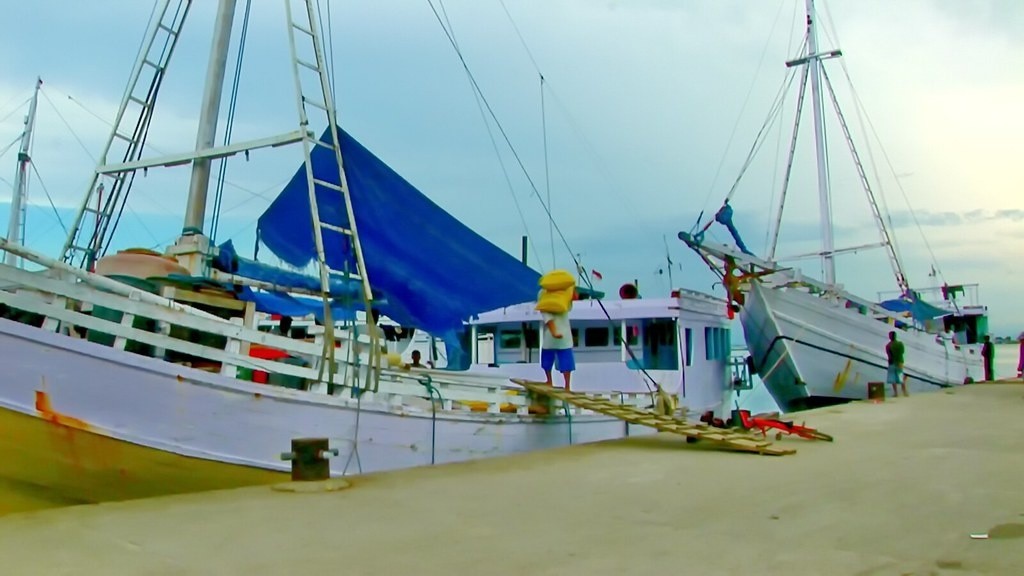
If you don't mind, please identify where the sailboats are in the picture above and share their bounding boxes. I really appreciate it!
[677,0,991,413]
[0,0,747,504]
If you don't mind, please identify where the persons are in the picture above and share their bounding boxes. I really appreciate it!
[981,336,995,382]
[405,350,427,369]
[269,316,293,338]
[535,271,578,392]
[885,331,908,397]
